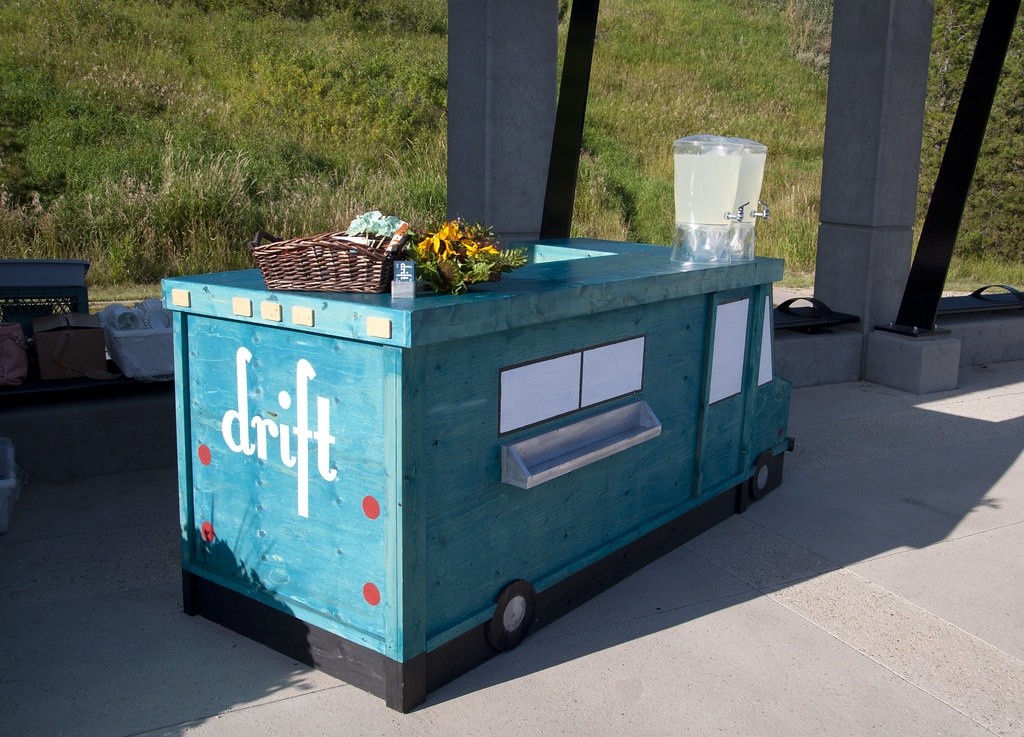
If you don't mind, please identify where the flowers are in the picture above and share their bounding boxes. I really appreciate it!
[386,210,529,298]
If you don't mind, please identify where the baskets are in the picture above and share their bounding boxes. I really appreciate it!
[0,438,15,533]
[247,230,415,293]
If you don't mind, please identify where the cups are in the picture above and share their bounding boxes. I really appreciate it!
[108,304,140,330]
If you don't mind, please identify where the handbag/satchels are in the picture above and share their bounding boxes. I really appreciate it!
[0,317,31,387]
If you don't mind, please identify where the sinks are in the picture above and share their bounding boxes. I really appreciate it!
[512,240,618,271]
[737,202,750,222]
[758,200,768,219]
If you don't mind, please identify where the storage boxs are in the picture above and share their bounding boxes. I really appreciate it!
[96,312,173,379]
[32,317,107,380]
